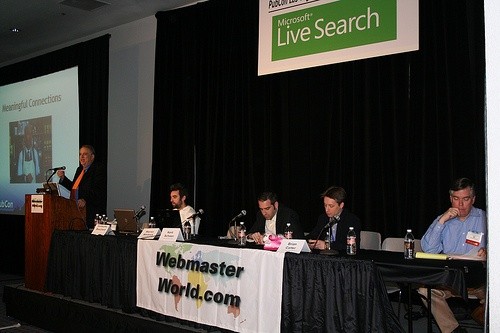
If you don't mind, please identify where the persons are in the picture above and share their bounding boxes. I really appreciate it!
[17,126,40,183]
[309,186,351,251]
[58,145,99,228]
[418,177,489,333]
[246,193,300,243]
[169,184,200,235]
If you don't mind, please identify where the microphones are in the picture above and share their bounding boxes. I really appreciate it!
[186,209,204,219]
[324,216,340,229]
[232,210,247,221]
[133,205,146,218]
[49,166,66,171]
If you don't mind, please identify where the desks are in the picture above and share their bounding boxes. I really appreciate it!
[44,228,487,333]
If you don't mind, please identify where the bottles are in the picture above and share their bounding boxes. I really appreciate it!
[346,227,356,255]
[404,230,416,259]
[94,214,108,227]
[183,220,191,241]
[148,217,155,228]
[238,222,246,245]
[284,224,293,239]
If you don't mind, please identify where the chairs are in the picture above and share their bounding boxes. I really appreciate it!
[360,231,489,333]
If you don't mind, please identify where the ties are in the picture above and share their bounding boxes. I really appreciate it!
[72,167,85,190]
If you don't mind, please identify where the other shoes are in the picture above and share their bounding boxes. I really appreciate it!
[454,328,467,333]
